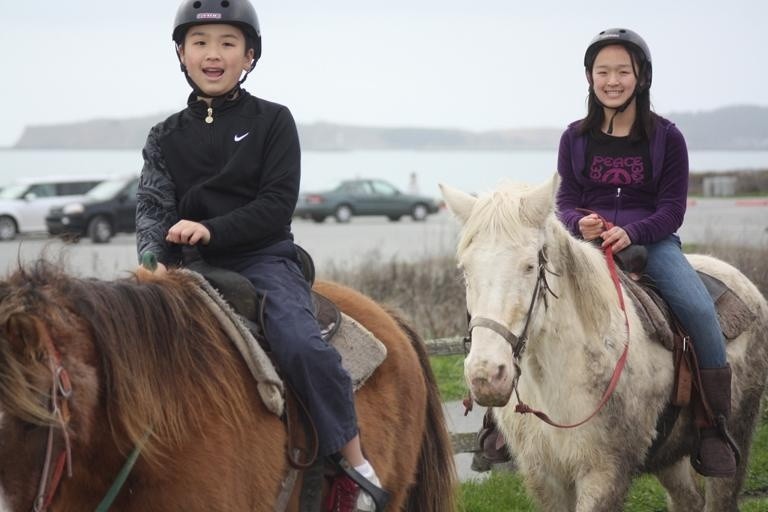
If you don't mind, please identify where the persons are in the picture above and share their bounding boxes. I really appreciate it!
[133,0,387,511]
[478,27,738,478]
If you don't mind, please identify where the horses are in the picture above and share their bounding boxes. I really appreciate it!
[0,227,463,512]
[436,171,768,512]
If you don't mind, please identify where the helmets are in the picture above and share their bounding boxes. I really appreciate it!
[584,28,653,94]
[172,0,262,63]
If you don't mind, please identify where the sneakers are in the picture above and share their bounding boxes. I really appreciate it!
[328,460,380,512]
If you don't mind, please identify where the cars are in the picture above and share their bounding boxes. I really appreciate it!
[44,176,140,244]
[0,174,111,241]
[291,179,446,223]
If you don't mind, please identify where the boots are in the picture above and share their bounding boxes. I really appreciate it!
[690,361,738,478]
[484,423,513,463]
